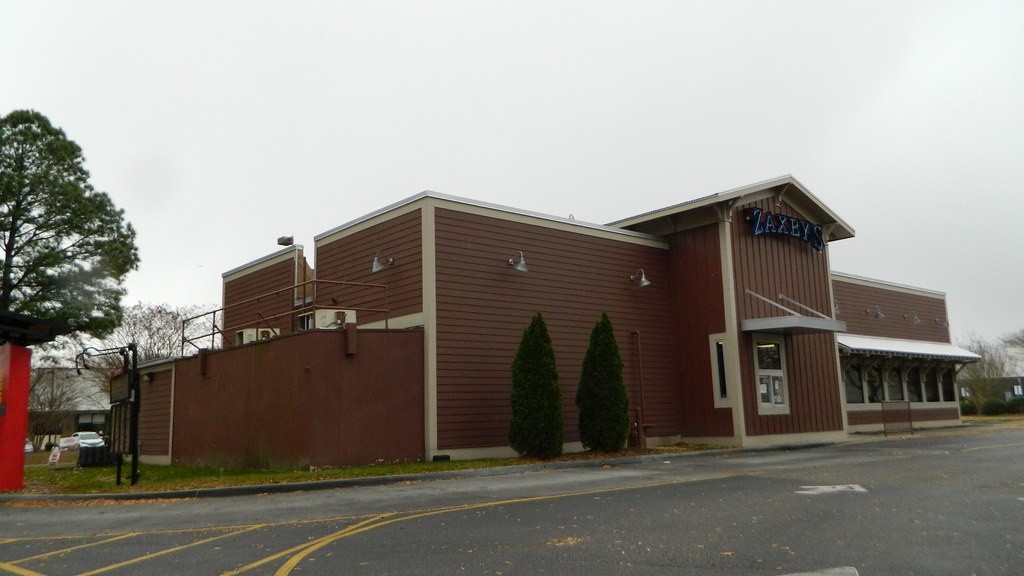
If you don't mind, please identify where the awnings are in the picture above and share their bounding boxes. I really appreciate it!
[836,336,981,383]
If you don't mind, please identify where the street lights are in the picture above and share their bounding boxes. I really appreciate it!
[76,343,140,485]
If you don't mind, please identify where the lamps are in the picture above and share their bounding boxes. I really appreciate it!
[867,305,885,319]
[509,249,529,272]
[372,250,394,273]
[903,311,921,324]
[934,316,949,327]
[629,268,652,287]
[833,298,840,314]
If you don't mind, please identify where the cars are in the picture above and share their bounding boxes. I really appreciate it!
[72,432,105,447]
[24,438,34,453]
